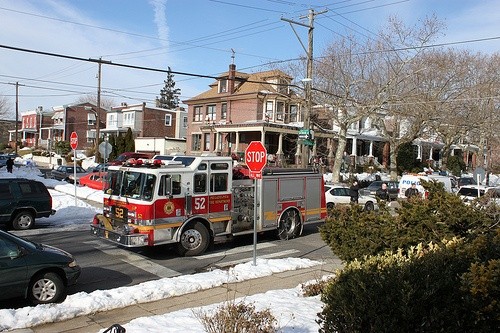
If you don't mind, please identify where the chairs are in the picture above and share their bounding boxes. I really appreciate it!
[173,182,180,194]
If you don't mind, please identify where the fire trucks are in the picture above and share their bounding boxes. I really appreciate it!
[89,154,328,255]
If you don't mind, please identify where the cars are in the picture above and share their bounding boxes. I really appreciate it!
[49,165,86,184]
[0,230,81,304]
[80,173,109,192]
[369,180,399,200]
[457,177,476,188]
[324,184,377,214]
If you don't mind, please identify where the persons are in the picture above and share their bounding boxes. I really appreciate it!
[405,183,419,199]
[230,152,246,162]
[123,181,155,197]
[6,156,14,173]
[308,155,335,166]
[349,179,359,205]
[376,183,391,210]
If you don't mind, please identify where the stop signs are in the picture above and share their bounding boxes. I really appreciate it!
[69,132,78,150]
[244,141,266,179]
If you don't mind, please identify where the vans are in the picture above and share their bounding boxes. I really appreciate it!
[398,175,452,200]
[455,184,500,210]
[0,178,56,229]
